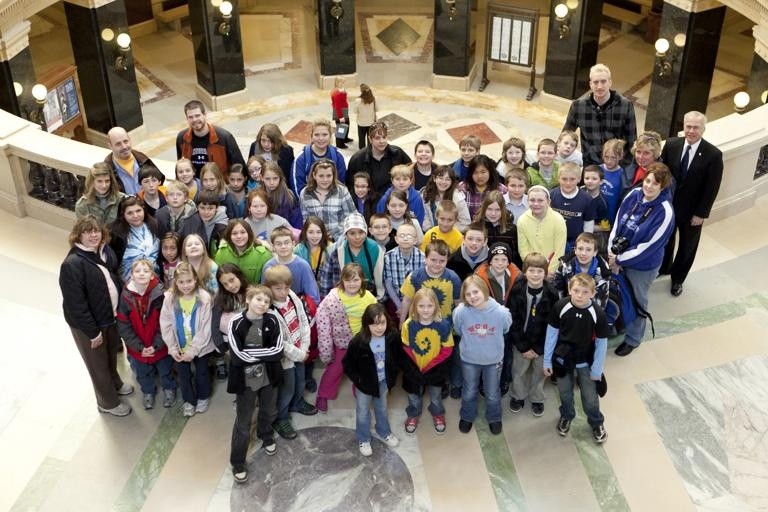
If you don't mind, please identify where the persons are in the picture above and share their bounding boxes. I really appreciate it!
[211,263,250,379]
[556,130,583,169]
[400,288,457,437]
[180,189,231,259]
[330,75,355,150]
[382,222,430,333]
[353,83,379,150]
[298,157,358,244]
[262,264,319,441]
[115,259,179,410]
[175,157,202,203]
[366,213,397,252]
[447,222,492,399]
[225,163,251,218]
[319,211,396,393]
[342,302,425,458]
[134,166,169,218]
[103,126,165,196]
[260,226,321,393]
[623,131,661,188]
[348,170,381,239]
[226,284,285,484]
[517,185,569,287]
[472,190,521,270]
[290,120,347,229]
[159,232,183,291]
[452,274,514,436]
[554,232,611,314]
[159,262,214,418]
[213,218,273,285]
[259,161,297,221]
[474,241,524,400]
[582,166,611,263]
[606,162,676,357]
[505,252,559,416]
[448,134,481,183]
[596,137,627,228]
[59,214,135,417]
[181,233,228,380]
[70,124,92,145]
[525,138,561,194]
[397,238,463,398]
[73,162,129,227]
[543,273,610,444]
[292,215,334,292]
[176,100,248,186]
[655,111,724,297]
[554,64,637,189]
[500,168,531,226]
[246,154,267,191]
[409,139,442,192]
[154,182,209,257]
[418,164,471,237]
[314,264,380,414]
[495,138,531,186]
[460,154,508,223]
[344,121,413,198]
[549,161,596,257]
[192,162,234,220]
[376,164,425,229]
[371,190,424,254]
[420,199,465,257]
[102,194,166,294]
[243,189,292,246]
[249,123,294,191]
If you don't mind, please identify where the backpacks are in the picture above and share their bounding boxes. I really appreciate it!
[605,271,655,340]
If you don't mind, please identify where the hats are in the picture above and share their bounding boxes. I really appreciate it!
[343,210,367,235]
[487,242,512,265]
[528,184,551,200]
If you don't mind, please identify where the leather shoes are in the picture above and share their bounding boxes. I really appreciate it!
[670,278,684,297]
[615,341,639,357]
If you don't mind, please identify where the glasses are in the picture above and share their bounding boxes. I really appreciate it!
[82,229,102,235]
[250,166,262,175]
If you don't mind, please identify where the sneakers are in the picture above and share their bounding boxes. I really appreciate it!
[97,402,132,417]
[143,392,155,410]
[376,430,400,447]
[305,379,317,392]
[591,422,608,443]
[557,415,571,437]
[231,464,248,483]
[316,392,328,414]
[405,417,419,433]
[216,361,227,382]
[162,387,177,407]
[439,385,447,399]
[500,383,509,395]
[433,416,446,435]
[262,439,277,456]
[297,400,317,416]
[489,419,502,435]
[116,382,135,395]
[451,386,462,398]
[196,397,211,413]
[359,439,372,457]
[478,386,486,399]
[532,402,545,417]
[272,418,297,439]
[458,419,471,433]
[510,397,524,413]
[182,402,196,416]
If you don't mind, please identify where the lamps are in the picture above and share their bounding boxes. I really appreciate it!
[30,84,49,126]
[734,90,752,115]
[554,3,571,42]
[653,37,674,82]
[113,33,137,72]
[216,1,233,37]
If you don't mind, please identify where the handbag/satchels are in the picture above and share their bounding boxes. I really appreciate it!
[334,120,349,140]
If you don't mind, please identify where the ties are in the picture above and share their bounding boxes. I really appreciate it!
[680,144,692,176]
